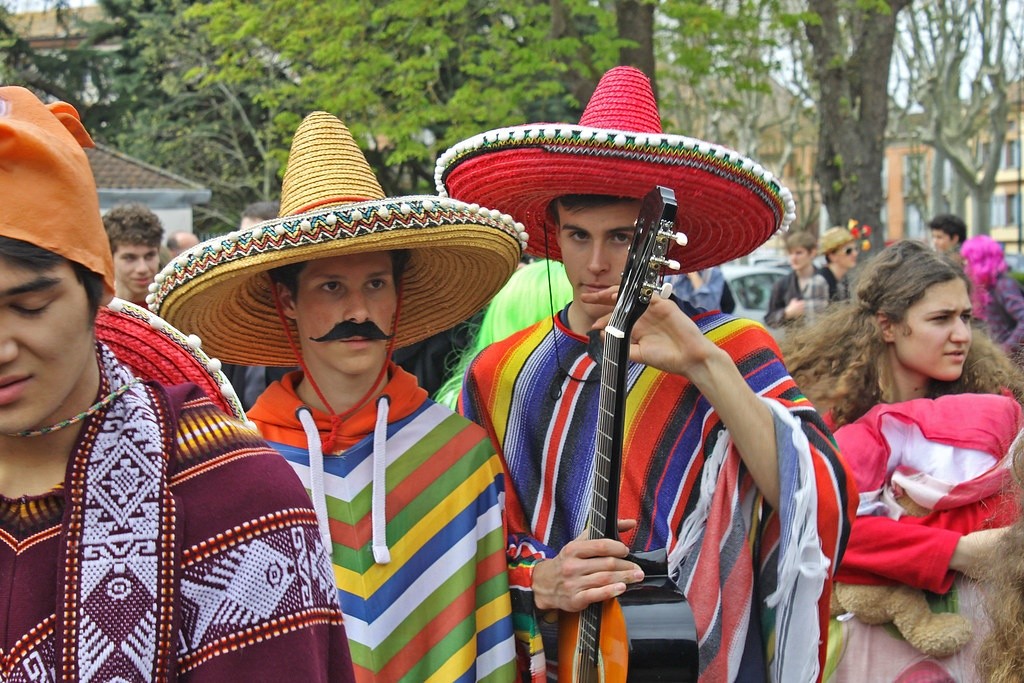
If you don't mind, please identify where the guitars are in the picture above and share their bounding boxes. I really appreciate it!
[555,186,700,683]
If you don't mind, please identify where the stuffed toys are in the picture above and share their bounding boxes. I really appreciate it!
[828,494,976,656]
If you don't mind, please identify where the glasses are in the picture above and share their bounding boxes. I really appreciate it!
[832,246,861,255]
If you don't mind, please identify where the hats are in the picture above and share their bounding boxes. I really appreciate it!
[435,66,796,281]
[0,87,116,304]
[816,227,853,255]
[146,111,528,368]
[96,296,254,432]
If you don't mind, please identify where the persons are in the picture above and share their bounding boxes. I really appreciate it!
[769,241,1024,683]
[665,269,726,311]
[100,196,163,309]
[167,231,201,259]
[434,65,859,683]
[961,233,1024,369]
[927,213,967,270]
[765,232,818,337]
[221,202,301,412]
[803,226,859,319]
[145,112,530,683]
[0,85,355,682]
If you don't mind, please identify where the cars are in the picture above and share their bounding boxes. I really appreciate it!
[719,253,796,342]
